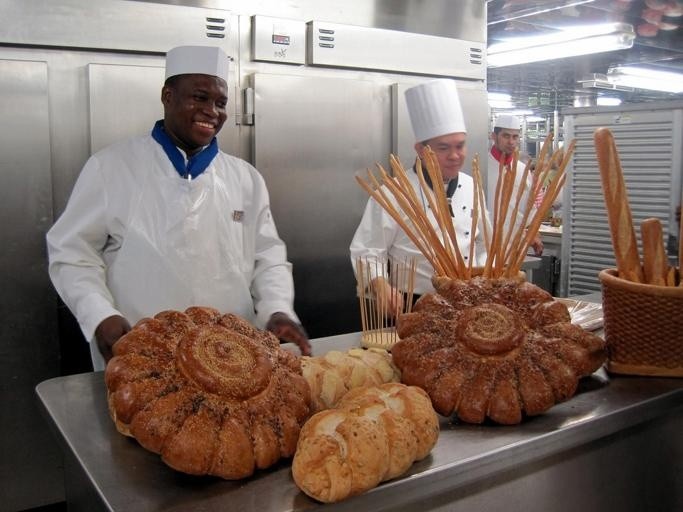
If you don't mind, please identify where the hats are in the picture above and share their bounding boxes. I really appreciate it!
[164,46,229,86]
[495,115,521,130]
[405,79,466,142]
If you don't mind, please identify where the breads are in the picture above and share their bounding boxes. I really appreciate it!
[291,383,441,503]
[665,267,679,285]
[392,274,611,427]
[300,347,402,413]
[105,307,315,481]
[640,218,668,286]
[594,128,645,285]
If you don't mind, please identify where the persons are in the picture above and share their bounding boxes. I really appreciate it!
[45,44,312,372]
[487,113,545,258]
[348,77,496,327]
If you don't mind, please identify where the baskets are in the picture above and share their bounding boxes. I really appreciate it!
[599,267,683,376]
